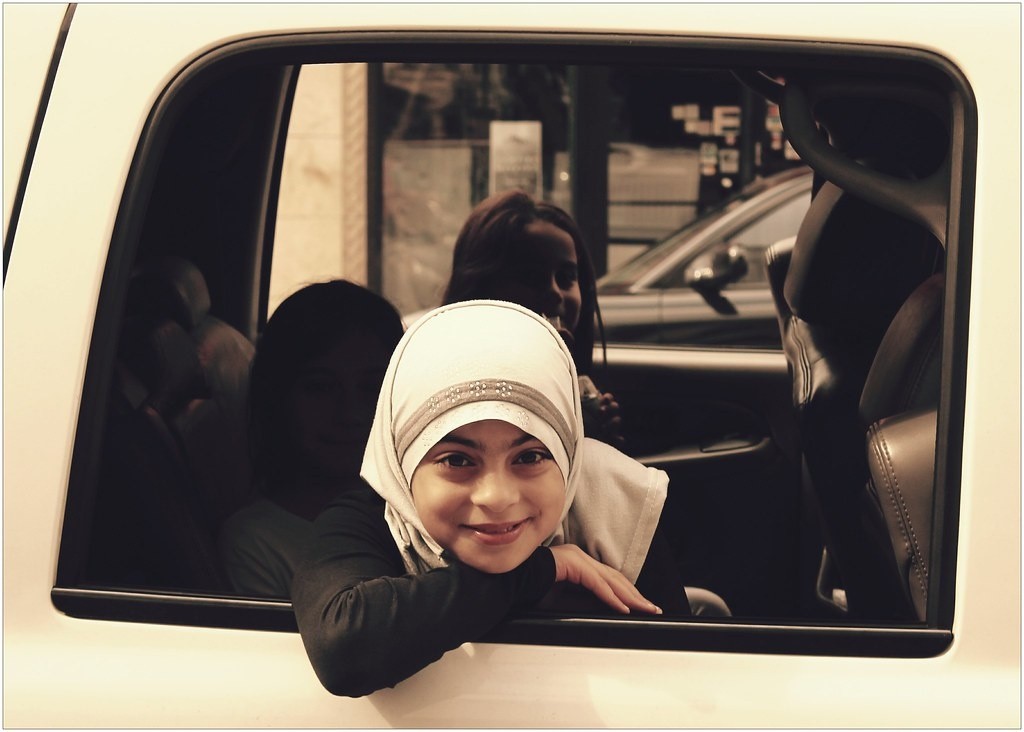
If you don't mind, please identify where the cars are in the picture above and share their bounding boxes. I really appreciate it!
[1,4,1022,728]
[594,159,817,350]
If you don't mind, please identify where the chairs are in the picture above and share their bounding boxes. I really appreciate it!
[782,180,947,634]
[101,240,260,592]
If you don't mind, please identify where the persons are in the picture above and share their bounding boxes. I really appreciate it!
[216,282,403,600]
[442,193,733,617]
[290,294,690,697]
